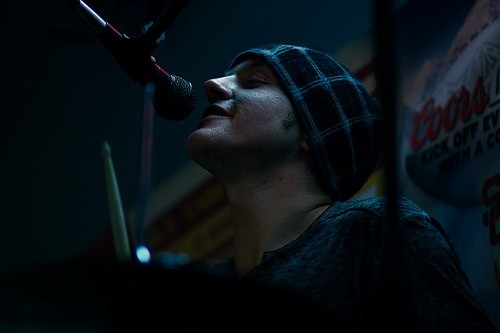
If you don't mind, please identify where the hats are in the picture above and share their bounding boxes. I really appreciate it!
[230,45,383,201]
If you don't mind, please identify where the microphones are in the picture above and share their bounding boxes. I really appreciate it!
[66,0,197,122]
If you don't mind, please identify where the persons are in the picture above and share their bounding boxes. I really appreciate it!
[129,43,494,333]
